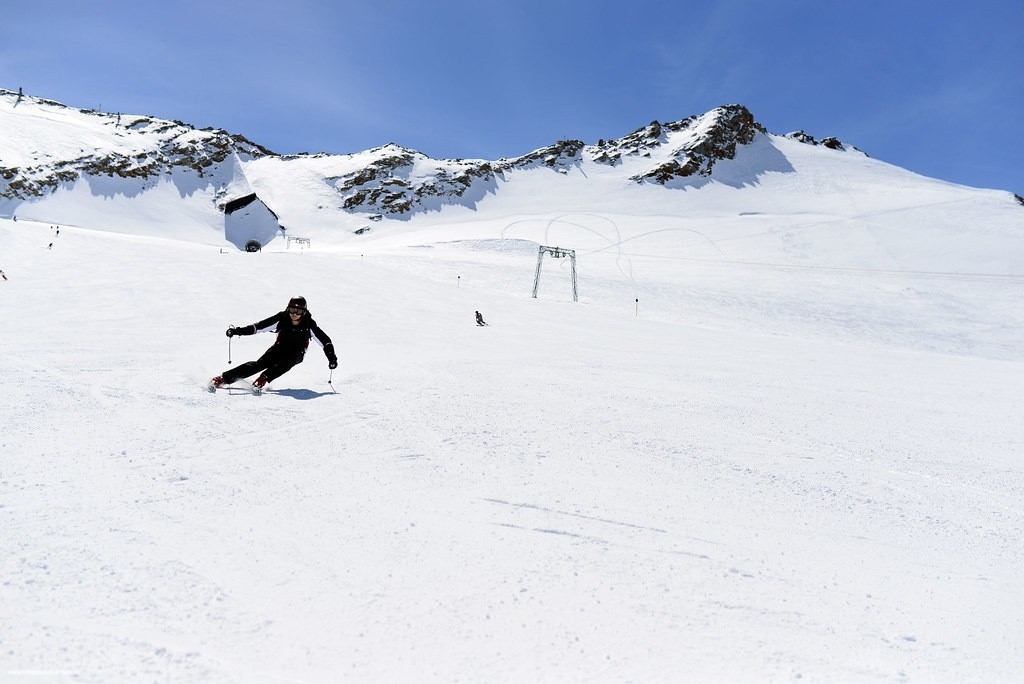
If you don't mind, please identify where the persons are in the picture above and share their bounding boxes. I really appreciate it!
[475,311,486,326]
[0,270,8,280]
[207,297,337,395]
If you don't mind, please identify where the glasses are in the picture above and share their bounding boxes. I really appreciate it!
[287,306,306,315]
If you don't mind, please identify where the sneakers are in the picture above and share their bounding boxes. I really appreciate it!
[252,374,267,389]
[212,374,224,388]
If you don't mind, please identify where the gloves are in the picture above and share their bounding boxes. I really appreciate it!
[323,344,338,369]
[226,324,257,337]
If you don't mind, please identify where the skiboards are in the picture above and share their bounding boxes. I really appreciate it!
[201,381,263,397]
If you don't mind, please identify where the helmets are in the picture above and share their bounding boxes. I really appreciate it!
[288,296,307,310]
[475,311,478,313]
